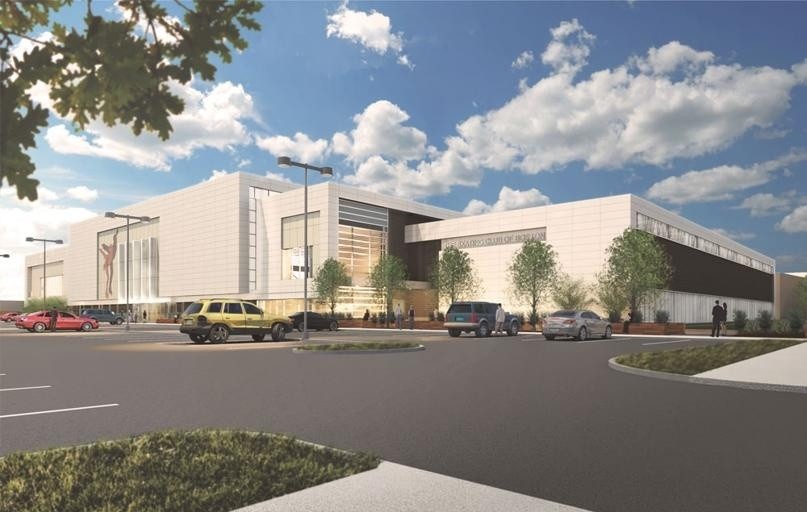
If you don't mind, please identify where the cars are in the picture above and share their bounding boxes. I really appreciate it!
[542,310,613,342]
[0,312,29,321]
[16,310,99,332]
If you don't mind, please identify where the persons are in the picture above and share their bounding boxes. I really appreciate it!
[407,304,415,330]
[432,306,438,321]
[111,308,147,323]
[363,309,370,321]
[626,313,631,321]
[98,233,118,298]
[711,299,723,337]
[721,302,727,336]
[493,303,505,336]
[49,306,58,331]
[395,304,403,330]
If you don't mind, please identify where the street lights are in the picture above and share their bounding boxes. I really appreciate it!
[103,211,150,330]
[25,236,63,308]
[277,155,334,342]
[0,254,10,258]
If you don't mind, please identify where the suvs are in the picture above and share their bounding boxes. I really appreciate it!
[178,298,294,344]
[81,308,125,325]
[288,311,339,332]
[443,300,522,339]
